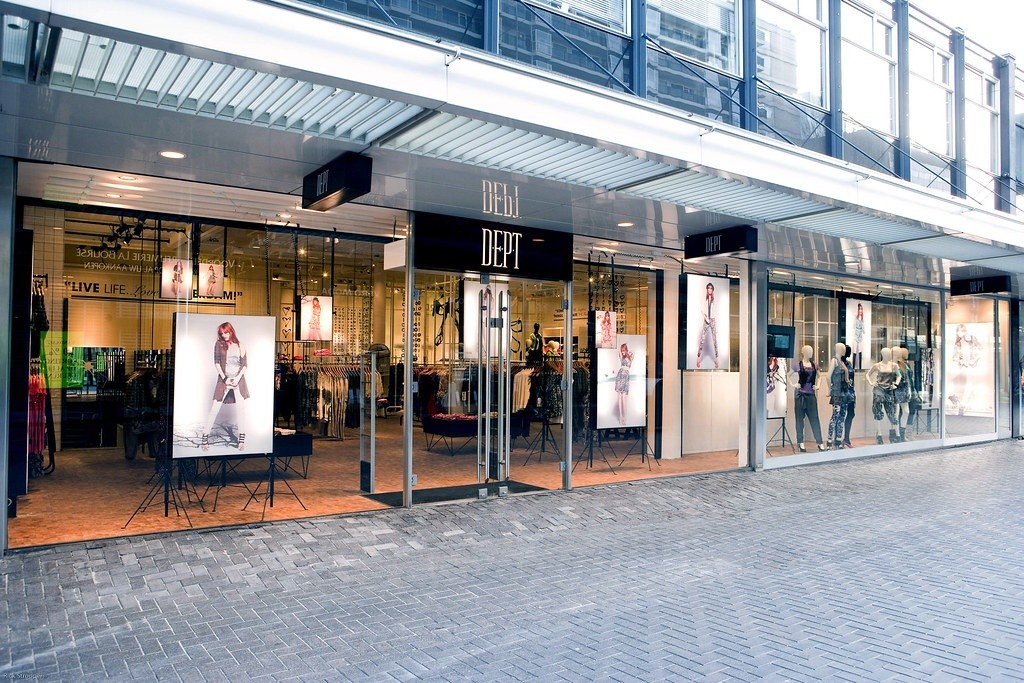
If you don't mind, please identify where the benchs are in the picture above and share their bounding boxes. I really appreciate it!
[194,430,313,487]
[423,411,499,458]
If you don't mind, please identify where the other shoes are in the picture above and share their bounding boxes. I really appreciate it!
[876,434,884,445]
[202,445,208,452]
[238,442,244,451]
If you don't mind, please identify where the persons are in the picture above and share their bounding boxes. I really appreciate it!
[614,343,634,425]
[948,324,982,410]
[890,346,919,441]
[853,303,865,369]
[786,345,825,452]
[207,265,217,296]
[482,285,494,357]
[172,260,183,297]
[865,348,902,445]
[202,322,251,452]
[697,283,719,368]
[826,343,856,451]
[307,297,322,340]
[601,311,612,348]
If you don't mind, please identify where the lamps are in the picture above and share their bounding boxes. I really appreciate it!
[77,216,146,253]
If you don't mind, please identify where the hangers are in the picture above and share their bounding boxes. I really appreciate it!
[31,274,45,297]
[29,359,45,384]
[297,358,588,377]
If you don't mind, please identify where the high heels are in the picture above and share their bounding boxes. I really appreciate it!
[798,443,806,452]
[818,445,825,451]
[827,438,855,449]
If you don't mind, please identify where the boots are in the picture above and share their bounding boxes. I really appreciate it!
[858,352,862,369]
[852,353,856,370]
[899,427,908,442]
[889,429,901,443]
